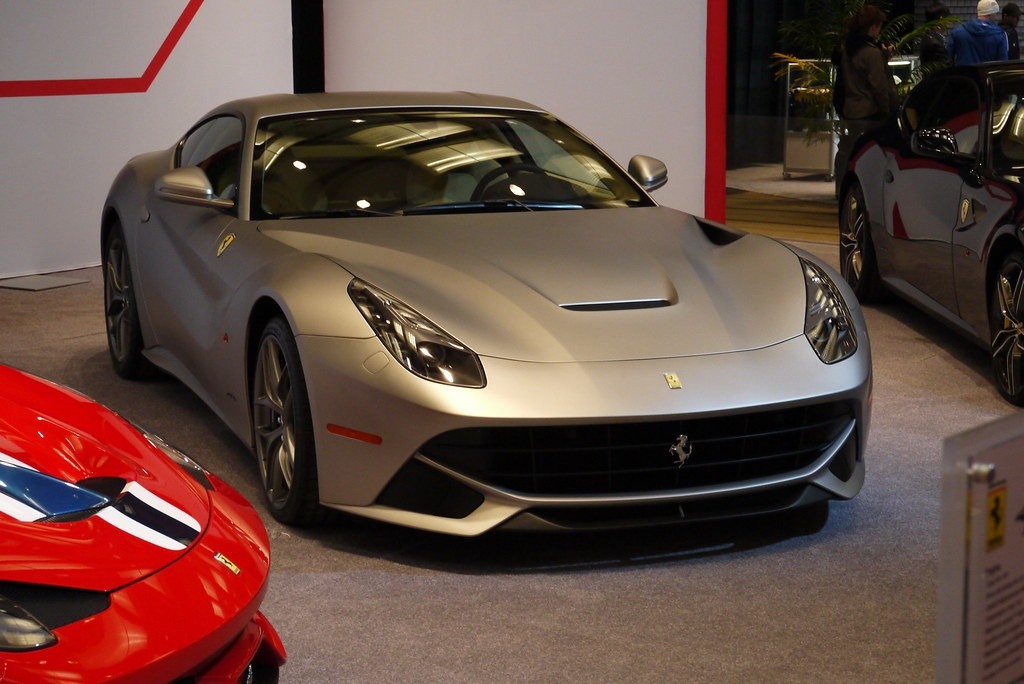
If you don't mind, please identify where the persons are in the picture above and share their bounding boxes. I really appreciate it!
[947,0,1009,65]
[998,2,1024,59]
[831,5,898,196]
[919,2,952,78]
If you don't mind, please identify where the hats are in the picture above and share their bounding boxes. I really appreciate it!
[977,0,999,16]
[1002,2,1024,17]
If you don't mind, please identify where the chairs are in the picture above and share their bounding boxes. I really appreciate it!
[263,157,321,214]
[406,155,477,205]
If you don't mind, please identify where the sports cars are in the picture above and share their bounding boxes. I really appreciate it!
[102,93,871,539]
[839,60,1024,408]
[0,364,287,684]
